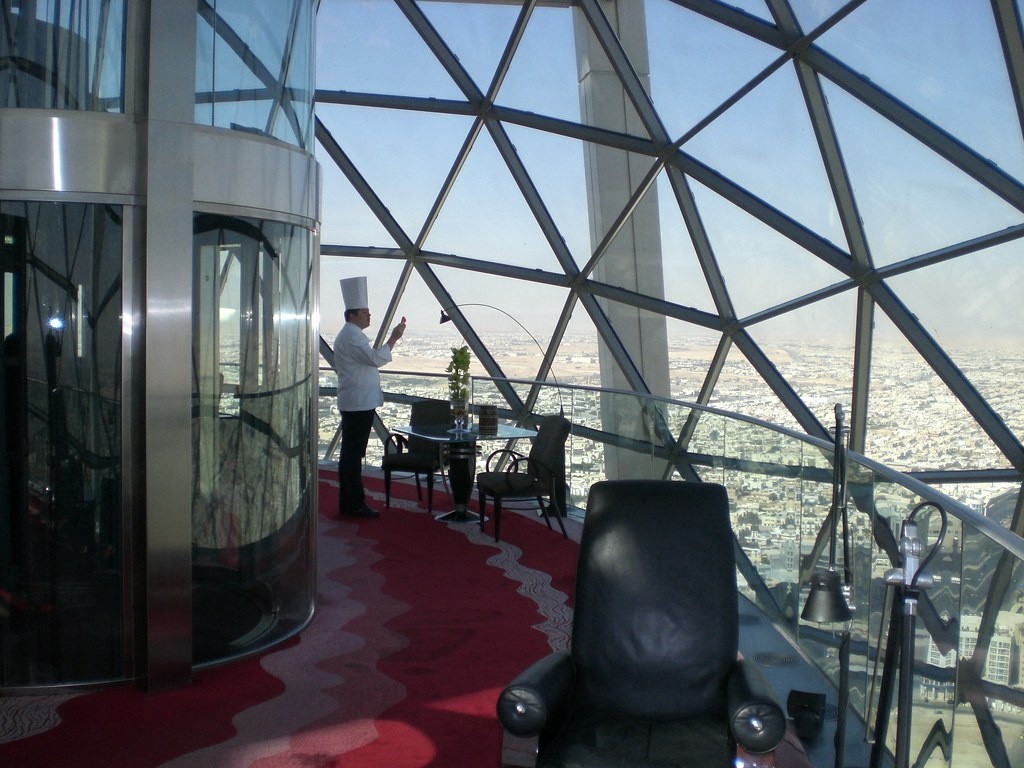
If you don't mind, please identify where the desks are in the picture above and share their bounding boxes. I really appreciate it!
[392,423,539,524]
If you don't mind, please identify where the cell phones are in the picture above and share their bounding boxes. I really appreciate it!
[401,317,406,326]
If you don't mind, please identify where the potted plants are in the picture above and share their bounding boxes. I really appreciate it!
[446,345,474,434]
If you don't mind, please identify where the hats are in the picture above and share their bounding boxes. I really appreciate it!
[340,276,368,310]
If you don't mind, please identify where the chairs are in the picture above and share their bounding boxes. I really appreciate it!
[477,416,572,543]
[496,478,786,768]
[381,401,451,513]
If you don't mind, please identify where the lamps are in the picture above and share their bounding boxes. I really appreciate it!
[799,403,857,768]
[441,303,566,418]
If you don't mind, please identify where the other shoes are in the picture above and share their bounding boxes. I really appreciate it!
[354,504,381,519]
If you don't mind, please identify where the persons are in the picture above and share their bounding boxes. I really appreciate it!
[334,277,406,519]
